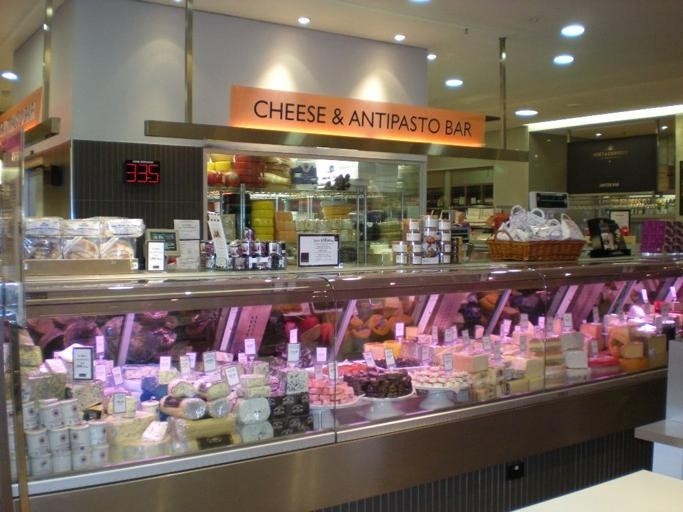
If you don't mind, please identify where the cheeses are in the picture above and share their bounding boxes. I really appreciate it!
[1,312,341,484]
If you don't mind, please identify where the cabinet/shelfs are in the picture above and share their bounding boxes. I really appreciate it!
[209,190,425,265]
[426,168,494,222]
[0,258,683,512]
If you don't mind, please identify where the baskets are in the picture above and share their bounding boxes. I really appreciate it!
[485,230,586,261]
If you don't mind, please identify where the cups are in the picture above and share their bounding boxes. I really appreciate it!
[8,432,16,454]
[24,427,49,457]
[10,461,17,483]
[41,402,65,430]
[39,398,58,425]
[53,449,72,474]
[22,400,38,430]
[69,424,90,445]
[141,400,160,420]
[60,399,82,425]
[49,428,70,450]
[26,456,30,476]
[72,447,92,471]
[6,401,14,433]
[30,453,53,476]
[92,444,110,465]
[89,421,106,446]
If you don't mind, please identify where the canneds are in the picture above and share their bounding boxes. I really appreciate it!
[201,228,287,271]
[390,214,461,265]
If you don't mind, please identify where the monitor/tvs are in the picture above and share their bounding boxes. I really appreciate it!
[565,133,659,198]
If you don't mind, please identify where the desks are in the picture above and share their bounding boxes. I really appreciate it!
[509,468,683,512]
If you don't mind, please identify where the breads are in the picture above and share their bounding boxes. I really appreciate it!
[342,317,531,404]
[24,213,146,264]
[531,300,683,394]
